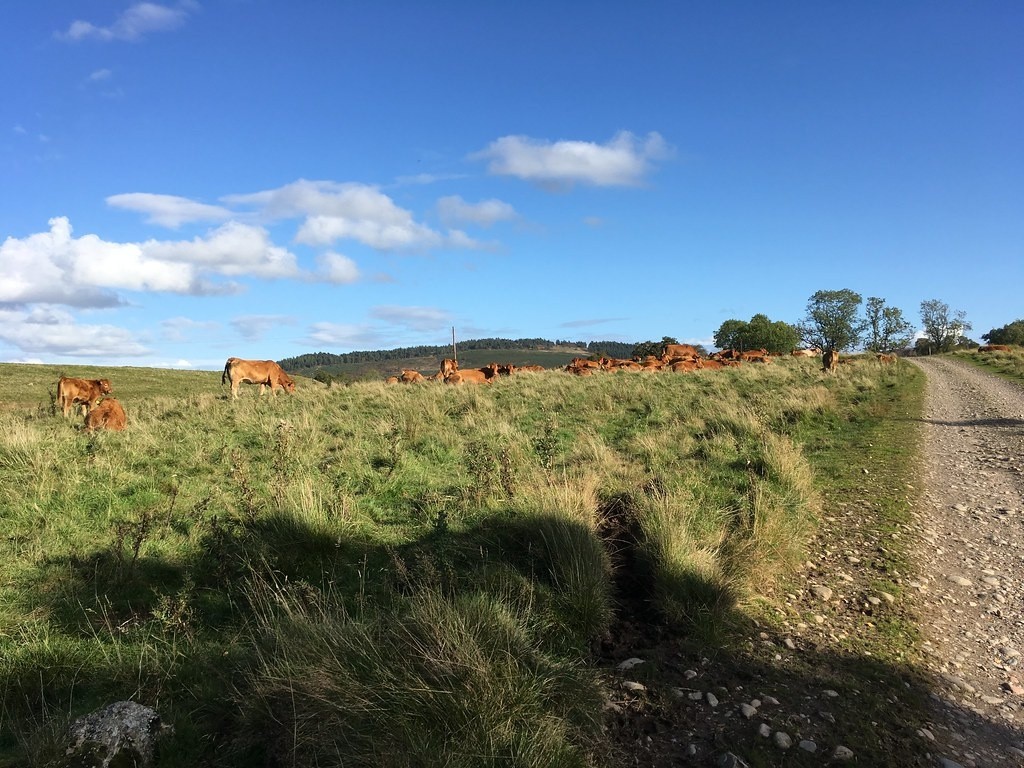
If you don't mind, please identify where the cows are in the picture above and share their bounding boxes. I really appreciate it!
[53,378,128,431]
[221,357,296,397]
[977,346,1013,353]
[386,344,894,384]
